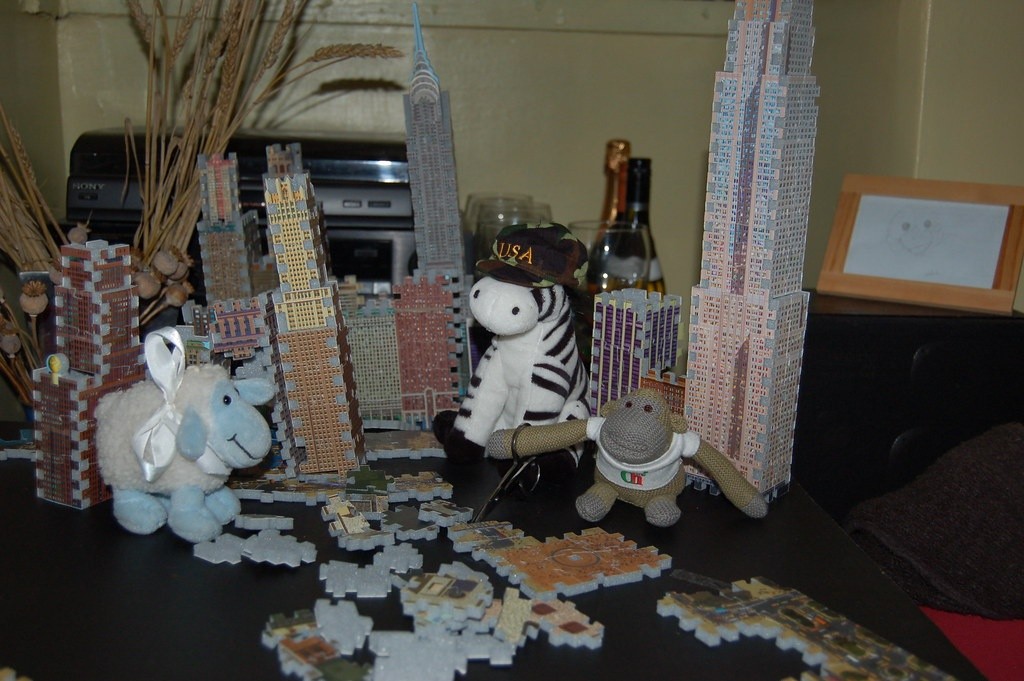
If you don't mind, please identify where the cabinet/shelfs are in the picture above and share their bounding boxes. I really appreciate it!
[792,289,1024,530]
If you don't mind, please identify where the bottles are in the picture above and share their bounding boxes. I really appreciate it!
[586,139,666,379]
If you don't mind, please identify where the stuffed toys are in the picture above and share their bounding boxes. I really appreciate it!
[487,389,769,527]
[432,219,589,499]
[93,327,277,543]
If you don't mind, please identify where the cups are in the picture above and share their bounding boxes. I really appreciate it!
[462,191,650,318]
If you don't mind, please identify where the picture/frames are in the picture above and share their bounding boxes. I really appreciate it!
[816,171,1024,316]
